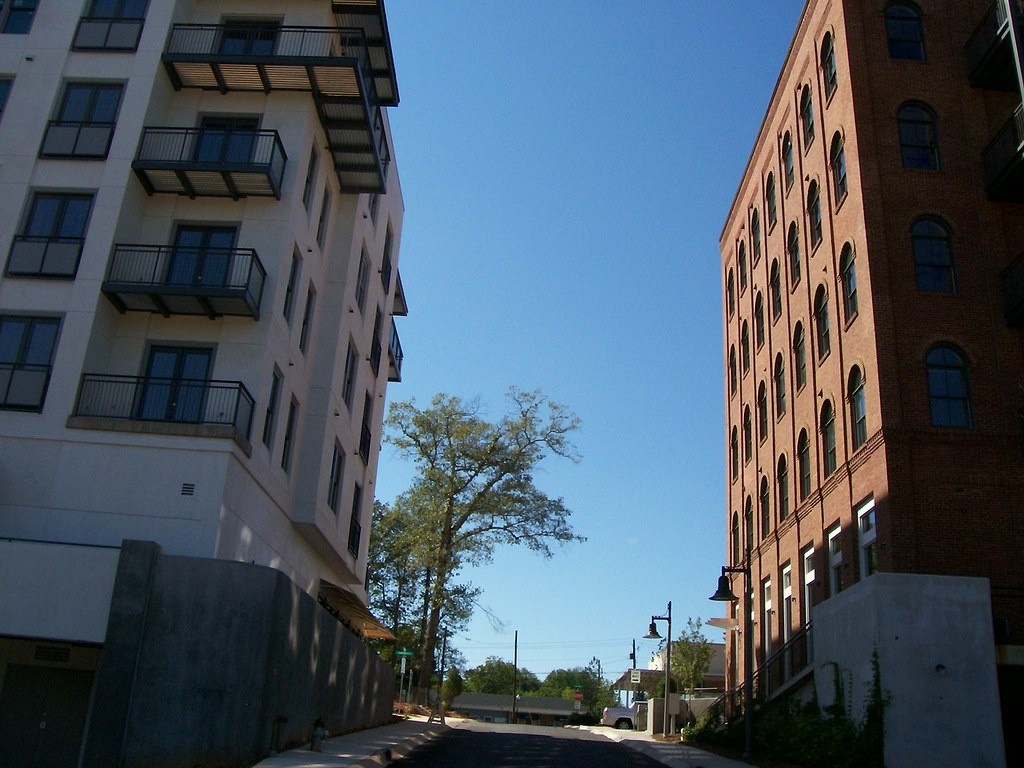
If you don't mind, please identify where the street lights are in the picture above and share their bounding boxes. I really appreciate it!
[709,544,753,760]
[642,601,671,738]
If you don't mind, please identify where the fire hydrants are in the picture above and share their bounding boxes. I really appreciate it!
[310,716,328,753]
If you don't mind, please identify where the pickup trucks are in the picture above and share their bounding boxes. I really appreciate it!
[600,701,649,730]
